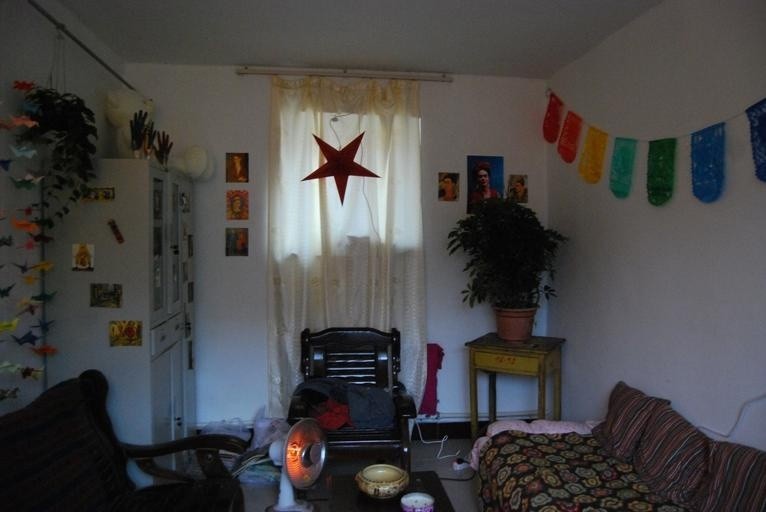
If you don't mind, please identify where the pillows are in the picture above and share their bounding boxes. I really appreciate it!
[632,403,710,503]
[690,440,766,512]
[590,381,670,461]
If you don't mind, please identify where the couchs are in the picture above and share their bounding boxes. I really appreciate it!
[472,418,765,512]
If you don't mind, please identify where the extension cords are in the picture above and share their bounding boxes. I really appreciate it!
[453,458,469,471]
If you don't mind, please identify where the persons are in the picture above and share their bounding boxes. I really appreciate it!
[513,180,527,203]
[471,163,499,200]
[441,174,457,199]
[230,155,247,181]
[143,121,157,157]
[152,132,174,172]
[127,109,149,158]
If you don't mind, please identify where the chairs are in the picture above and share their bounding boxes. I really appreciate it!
[286,326,417,472]
[0,368,251,512]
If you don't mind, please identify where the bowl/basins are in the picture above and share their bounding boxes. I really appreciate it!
[400,493,436,512]
[353,464,411,500]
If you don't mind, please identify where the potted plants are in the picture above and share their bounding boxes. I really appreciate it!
[446,196,571,346]
[0,79,99,183]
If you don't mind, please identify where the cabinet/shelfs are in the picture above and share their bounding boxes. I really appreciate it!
[40,158,197,480]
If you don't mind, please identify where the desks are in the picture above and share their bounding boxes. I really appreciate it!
[465,332,566,443]
[182,470,455,512]
